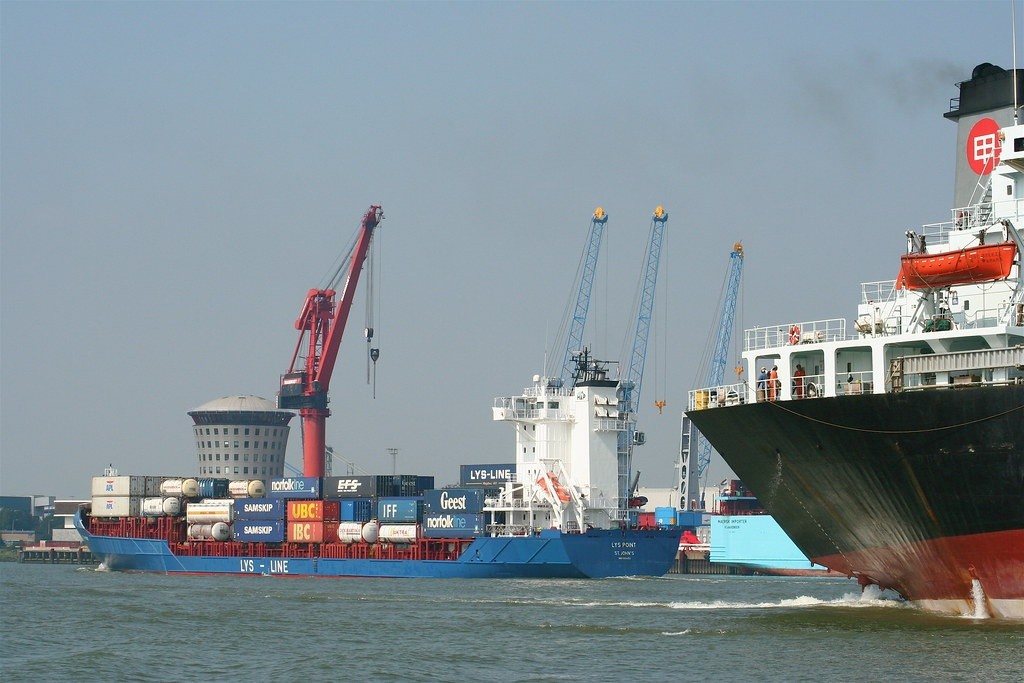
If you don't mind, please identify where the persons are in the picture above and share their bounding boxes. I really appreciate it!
[793,363,806,399]
[757,365,779,401]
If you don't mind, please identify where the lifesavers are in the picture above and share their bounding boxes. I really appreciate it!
[789,326,799,344]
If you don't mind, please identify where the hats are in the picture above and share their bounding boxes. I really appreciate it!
[761,367,766,370]
[796,364,801,367]
[772,365,777,370]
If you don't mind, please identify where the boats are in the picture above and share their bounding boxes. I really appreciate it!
[683,1,1023,621]
[72,340,685,580]
[896,242,1017,292]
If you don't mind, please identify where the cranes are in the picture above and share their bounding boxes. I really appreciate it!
[613,204,675,527]
[275,200,385,471]
[654,238,746,527]
[538,205,608,394]
